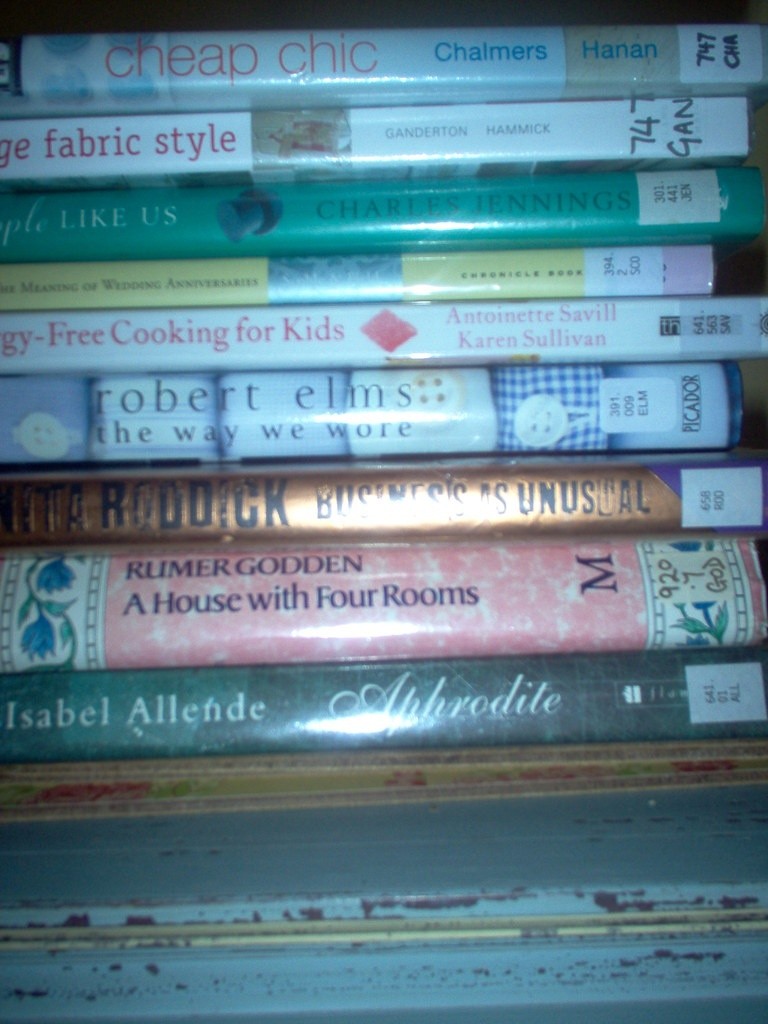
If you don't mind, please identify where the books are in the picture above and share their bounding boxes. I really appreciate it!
[0,96,756,192]
[0,166,766,264]
[0,646,768,762]
[0,244,714,312]
[0,456,768,546]
[0,360,744,463]
[20,24,768,119]
[0,536,768,674]
[0,296,768,375]
